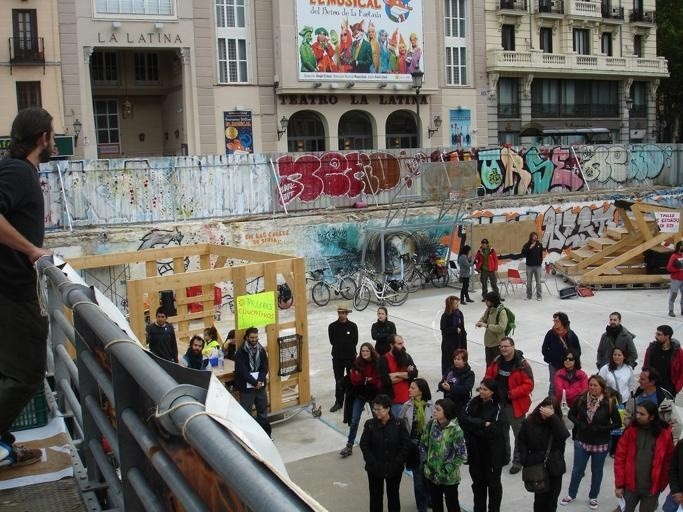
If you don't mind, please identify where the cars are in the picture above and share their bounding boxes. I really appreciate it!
[306,267,358,307]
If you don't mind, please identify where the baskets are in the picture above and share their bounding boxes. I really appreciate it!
[11,383,48,432]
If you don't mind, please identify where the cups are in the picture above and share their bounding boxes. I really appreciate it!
[208,358,217,366]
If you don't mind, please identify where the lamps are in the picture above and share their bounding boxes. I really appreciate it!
[119,51,134,119]
[427,116,442,138]
[72,119,81,147]
[276,116,288,140]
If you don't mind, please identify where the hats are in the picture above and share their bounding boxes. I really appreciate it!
[485,291,499,300]
[335,303,353,313]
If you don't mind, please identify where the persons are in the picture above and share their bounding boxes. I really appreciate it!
[299,20,421,75]
[0,107,57,471]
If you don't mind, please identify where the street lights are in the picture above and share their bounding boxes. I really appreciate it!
[410,62,424,148]
[625,95,633,144]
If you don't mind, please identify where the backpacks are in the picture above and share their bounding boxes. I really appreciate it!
[486,307,515,336]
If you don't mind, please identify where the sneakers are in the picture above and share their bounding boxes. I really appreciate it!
[460,294,542,305]
[669,309,675,317]
[330,401,343,412]
[1,444,42,470]
[340,446,352,457]
[589,498,599,509]
[560,494,576,506]
[509,465,520,475]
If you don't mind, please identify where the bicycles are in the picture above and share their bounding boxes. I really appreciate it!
[352,253,448,311]
[276,283,292,310]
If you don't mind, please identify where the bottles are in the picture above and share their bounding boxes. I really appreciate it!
[216,347,225,371]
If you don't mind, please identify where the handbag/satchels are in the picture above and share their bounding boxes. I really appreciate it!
[609,404,627,437]
[558,389,575,429]
[405,443,421,470]
[522,465,549,491]
